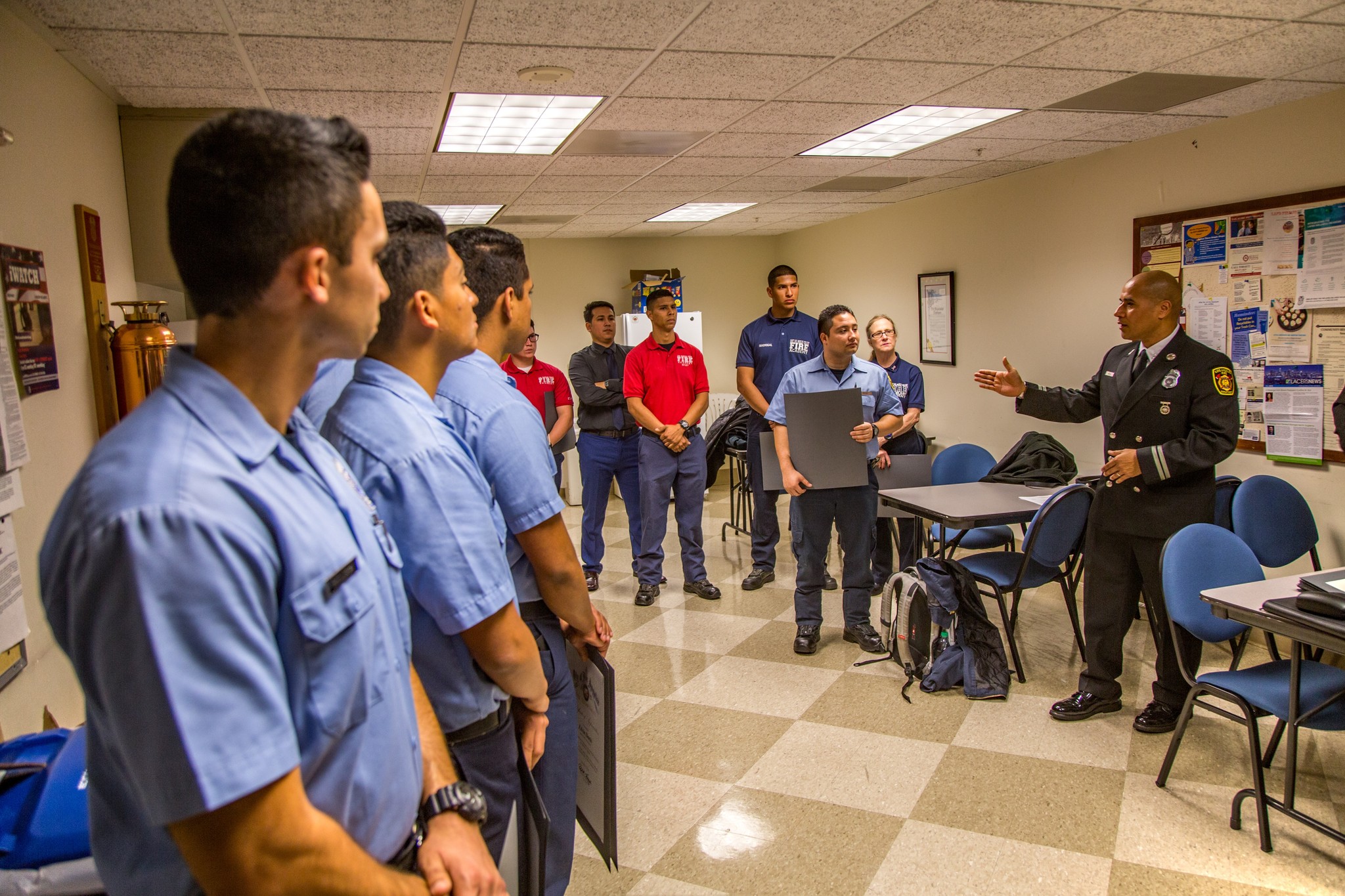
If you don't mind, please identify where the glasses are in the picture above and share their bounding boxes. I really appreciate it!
[525,333,539,343]
[870,329,895,339]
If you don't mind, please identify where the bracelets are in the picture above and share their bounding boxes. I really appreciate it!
[1016,380,1029,399]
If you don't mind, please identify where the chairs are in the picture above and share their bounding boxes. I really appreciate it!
[947,483,1097,681]
[1148,522,1344,857]
[698,392,744,446]
[925,442,1018,576]
[1226,474,1344,772]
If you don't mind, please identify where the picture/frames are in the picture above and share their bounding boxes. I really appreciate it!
[915,270,956,366]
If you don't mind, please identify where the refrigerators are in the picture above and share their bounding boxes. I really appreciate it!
[611,310,710,501]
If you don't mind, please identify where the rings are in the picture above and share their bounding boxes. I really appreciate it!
[1115,472,1122,477]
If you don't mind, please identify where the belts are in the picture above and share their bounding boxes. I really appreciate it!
[443,694,512,744]
[518,599,556,622]
[580,425,639,438]
[391,824,426,875]
[642,424,701,439]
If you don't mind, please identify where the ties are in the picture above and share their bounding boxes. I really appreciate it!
[1131,349,1148,386]
[605,348,625,431]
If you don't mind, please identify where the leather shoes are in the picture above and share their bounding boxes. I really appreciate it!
[871,582,885,596]
[633,571,667,582]
[683,578,722,599]
[1132,698,1194,733]
[635,583,660,605]
[584,571,599,590]
[1049,691,1123,721]
[842,621,882,652]
[823,565,838,590]
[741,567,775,590]
[793,625,821,654]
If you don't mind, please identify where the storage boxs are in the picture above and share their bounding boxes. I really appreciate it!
[620,266,686,315]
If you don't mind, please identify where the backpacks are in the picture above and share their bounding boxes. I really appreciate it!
[880,565,960,681]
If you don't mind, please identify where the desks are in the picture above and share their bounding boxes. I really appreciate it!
[1198,565,1345,850]
[877,477,1146,639]
[718,447,762,551]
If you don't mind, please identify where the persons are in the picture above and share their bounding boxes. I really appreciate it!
[319,196,566,896]
[629,288,725,606]
[969,268,1244,736]
[561,300,670,596]
[765,303,906,656]
[494,317,573,494]
[862,314,926,470]
[34,104,511,895]
[443,220,617,892]
[290,200,444,436]
[735,264,837,591]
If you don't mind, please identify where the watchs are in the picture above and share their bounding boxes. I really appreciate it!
[870,423,880,439]
[604,379,610,390]
[677,419,690,431]
[415,779,492,830]
[884,434,893,440]
